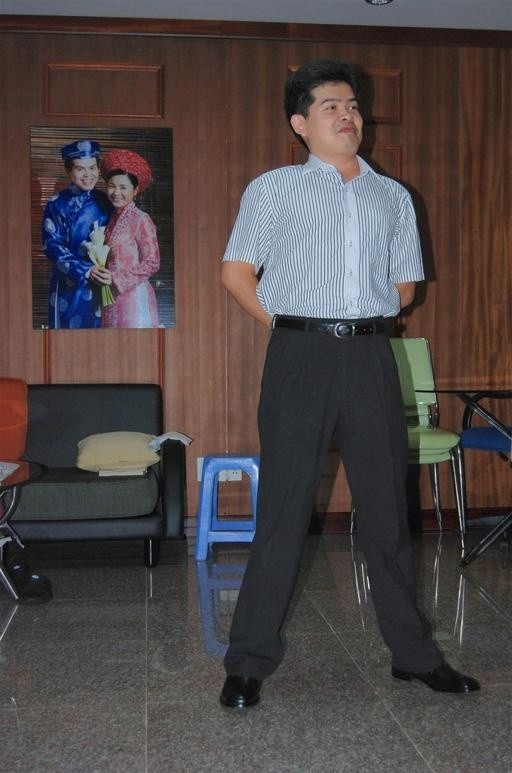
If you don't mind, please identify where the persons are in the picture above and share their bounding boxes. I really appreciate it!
[101,149,161,329]
[220,60,481,708]
[42,141,113,329]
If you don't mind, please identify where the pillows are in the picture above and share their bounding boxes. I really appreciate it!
[75,430,160,473]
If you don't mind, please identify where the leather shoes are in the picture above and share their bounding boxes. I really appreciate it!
[391,662,480,694]
[221,675,260,707]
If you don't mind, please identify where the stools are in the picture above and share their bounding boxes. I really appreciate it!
[195,454,260,562]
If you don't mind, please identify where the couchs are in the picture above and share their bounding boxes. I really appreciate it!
[6,383,187,568]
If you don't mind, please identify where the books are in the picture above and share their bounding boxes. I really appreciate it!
[99,469,147,477]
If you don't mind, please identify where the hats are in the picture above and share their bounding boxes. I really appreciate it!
[60,141,99,161]
[100,149,152,195]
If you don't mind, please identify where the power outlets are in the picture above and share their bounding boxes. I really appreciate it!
[196,457,241,483]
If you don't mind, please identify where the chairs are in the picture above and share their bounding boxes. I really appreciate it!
[0,378,31,602]
[349,338,469,557]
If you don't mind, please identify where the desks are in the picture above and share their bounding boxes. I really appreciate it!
[413,378,512,565]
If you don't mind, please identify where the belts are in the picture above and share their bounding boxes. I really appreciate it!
[275,318,383,338]
